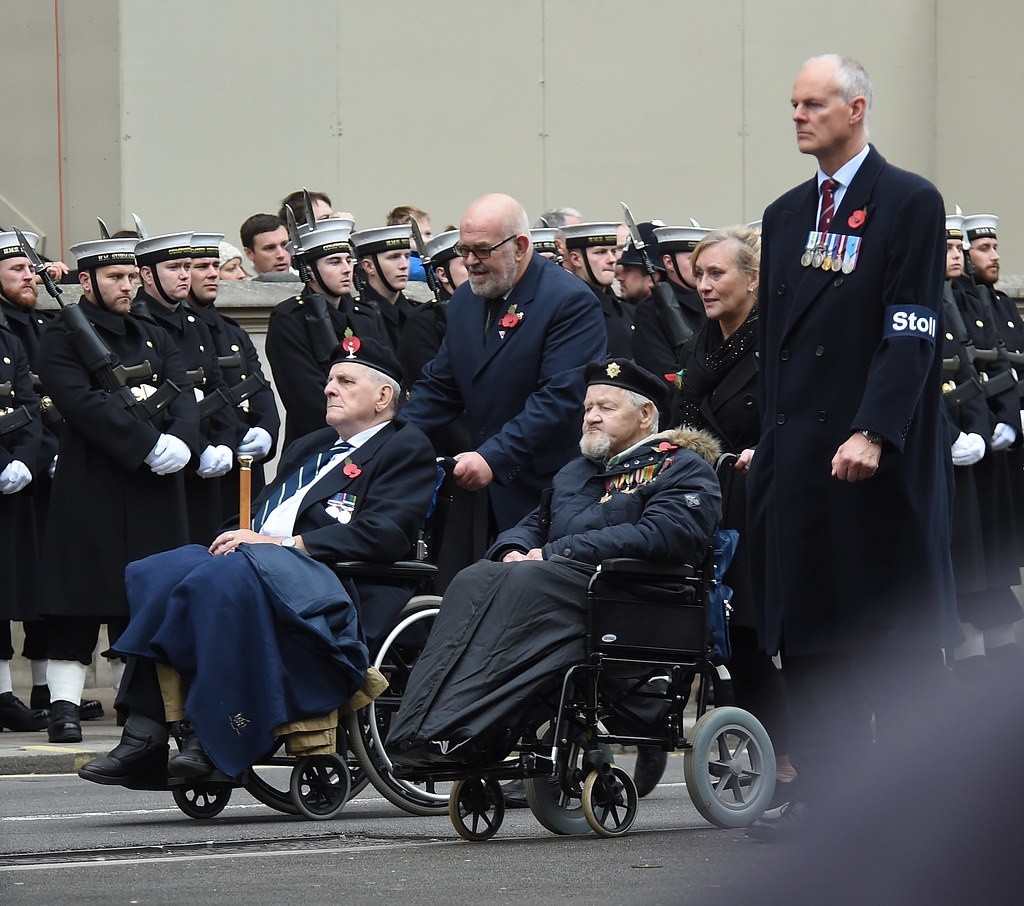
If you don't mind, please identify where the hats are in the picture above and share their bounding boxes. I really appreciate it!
[526,228,562,256]
[581,356,670,413]
[743,220,764,236]
[351,225,413,258]
[964,214,1000,240]
[652,225,726,253]
[560,221,630,247]
[0,231,40,261]
[219,241,242,270]
[616,221,668,271]
[285,227,351,262]
[296,217,355,236]
[190,233,226,262]
[135,231,196,268]
[329,337,403,386]
[943,215,964,241]
[69,237,140,272]
[425,229,465,270]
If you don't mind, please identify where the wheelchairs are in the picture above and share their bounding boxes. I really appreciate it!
[120,457,524,823]
[393,451,775,843]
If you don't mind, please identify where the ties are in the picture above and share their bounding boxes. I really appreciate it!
[818,178,841,241]
[483,297,504,348]
[250,439,353,534]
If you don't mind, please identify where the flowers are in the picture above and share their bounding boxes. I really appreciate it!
[848,206,867,228]
[503,304,519,326]
[343,457,362,478]
[665,369,687,389]
[343,326,361,353]
[651,441,677,454]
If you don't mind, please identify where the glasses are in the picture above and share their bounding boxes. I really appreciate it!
[452,234,517,259]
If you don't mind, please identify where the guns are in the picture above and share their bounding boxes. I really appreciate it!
[133,212,266,410]
[285,202,339,373]
[539,215,566,266]
[11,224,183,434]
[0,371,33,438]
[303,187,385,343]
[689,218,703,229]
[27,363,66,425]
[96,215,229,421]
[620,200,689,363]
[409,214,449,334]
[940,200,1024,413]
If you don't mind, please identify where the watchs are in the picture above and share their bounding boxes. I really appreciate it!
[856,429,885,445]
[280,536,297,548]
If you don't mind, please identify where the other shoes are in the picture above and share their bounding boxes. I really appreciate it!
[29,684,105,720]
[743,795,816,843]
[765,776,801,811]
[391,735,471,769]
[497,774,539,808]
[47,699,83,743]
[78,724,170,786]
[0,691,50,733]
[630,726,674,797]
[177,743,216,779]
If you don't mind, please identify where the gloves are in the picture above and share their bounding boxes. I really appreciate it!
[0,460,33,495]
[948,431,985,467]
[196,444,233,478]
[991,422,1018,451]
[238,426,272,461]
[145,431,191,476]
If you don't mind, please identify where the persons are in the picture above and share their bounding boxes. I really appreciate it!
[387,357,722,769]
[0,191,1024,799]
[80,340,435,790]
[746,56,963,844]
